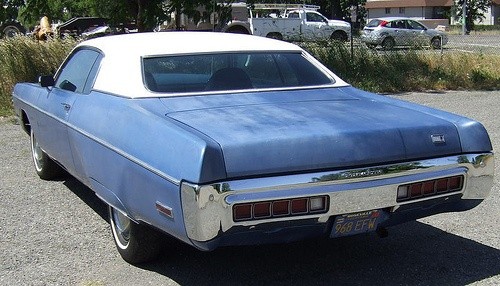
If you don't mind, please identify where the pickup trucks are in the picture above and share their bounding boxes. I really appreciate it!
[247,3,352,44]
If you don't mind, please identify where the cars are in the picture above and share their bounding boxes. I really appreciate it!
[12,32,494,268]
[361,16,448,51]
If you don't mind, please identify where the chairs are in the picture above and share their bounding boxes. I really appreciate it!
[203,67,253,92]
